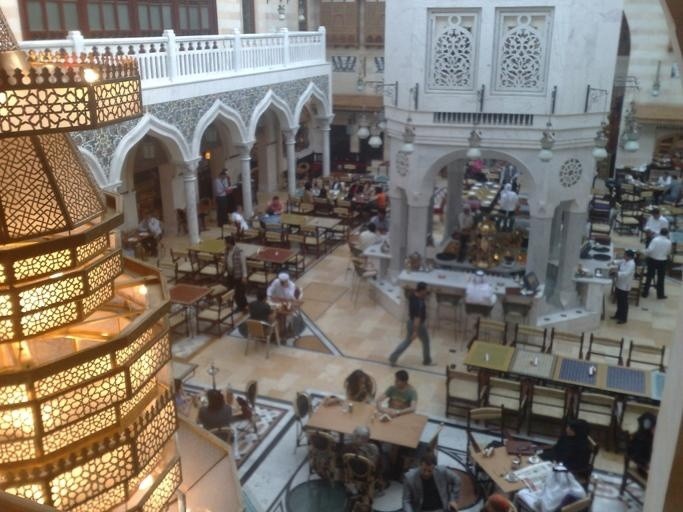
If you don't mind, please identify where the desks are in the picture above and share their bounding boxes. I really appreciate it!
[305,395,426,472]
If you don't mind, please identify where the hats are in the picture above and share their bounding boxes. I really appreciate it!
[278,272,289,282]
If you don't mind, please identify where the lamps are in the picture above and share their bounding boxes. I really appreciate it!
[593,90,611,159]
[354,79,385,150]
[467,84,485,162]
[397,83,419,155]
[2,8,187,512]
[624,89,642,151]
[538,90,557,161]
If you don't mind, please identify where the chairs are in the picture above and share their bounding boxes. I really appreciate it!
[443,320,665,439]
[211,427,234,444]
[618,438,652,505]
[436,405,597,511]
[121,225,156,259]
[156,178,386,339]
[239,383,261,431]
[401,286,533,349]
[289,369,378,502]
[611,174,683,306]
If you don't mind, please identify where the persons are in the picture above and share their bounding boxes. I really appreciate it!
[137,211,163,257]
[292,178,388,207]
[386,281,437,368]
[466,270,494,308]
[608,162,683,324]
[627,413,656,478]
[501,182,518,231]
[346,426,385,468]
[175,378,231,429]
[265,196,284,215]
[455,204,476,231]
[343,369,417,414]
[214,168,253,236]
[401,449,458,512]
[521,418,591,512]
[356,208,389,250]
[245,272,300,329]
[222,236,248,310]
